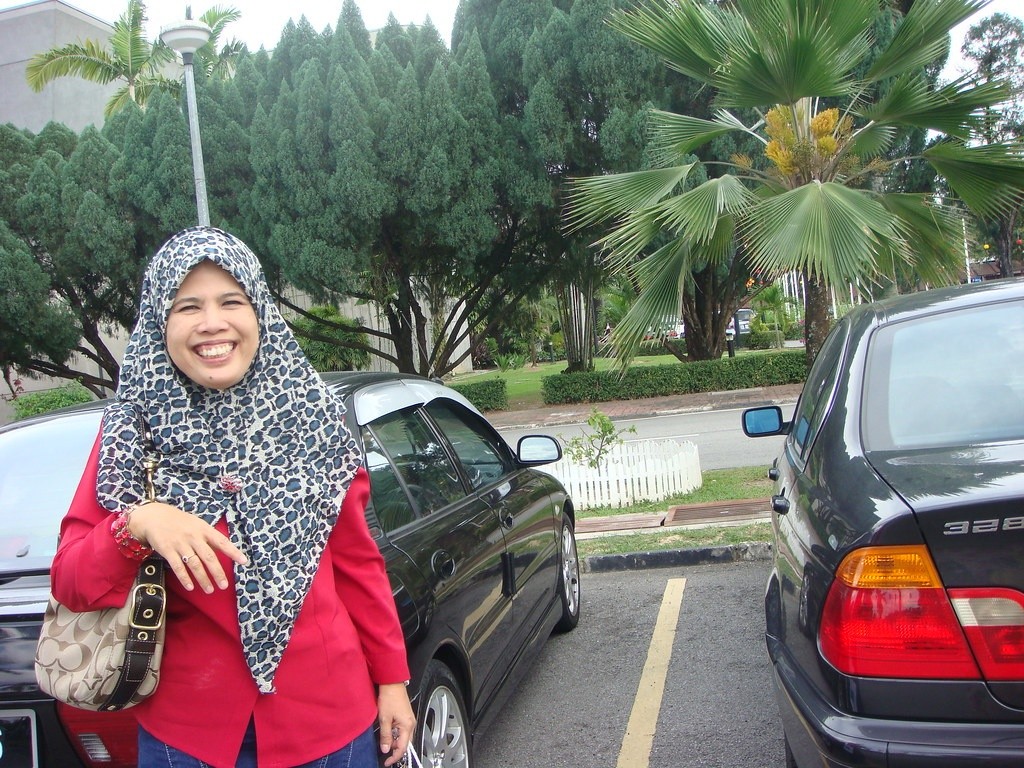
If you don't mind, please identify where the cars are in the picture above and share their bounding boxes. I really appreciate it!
[738,279,1024,768]
[1,371,581,768]
[643,306,758,343]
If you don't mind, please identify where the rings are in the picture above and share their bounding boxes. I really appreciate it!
[183,554,197,563]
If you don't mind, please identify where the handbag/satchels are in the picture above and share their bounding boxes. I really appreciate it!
[33,413,168,711]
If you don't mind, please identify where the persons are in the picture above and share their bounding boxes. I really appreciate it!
[50,226,417,768]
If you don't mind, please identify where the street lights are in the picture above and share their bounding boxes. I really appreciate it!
[160,17,213,226]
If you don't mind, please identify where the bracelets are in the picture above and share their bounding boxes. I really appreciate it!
[404,680,410,686]
[110,498,156,560]
